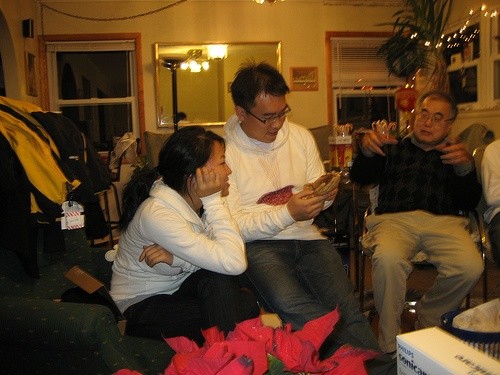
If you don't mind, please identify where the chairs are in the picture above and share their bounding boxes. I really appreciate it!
[310,120,495,312]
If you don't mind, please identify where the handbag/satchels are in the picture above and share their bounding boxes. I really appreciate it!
[314,186,370,236]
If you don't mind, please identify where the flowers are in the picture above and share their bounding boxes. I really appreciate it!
[112,302,381,375]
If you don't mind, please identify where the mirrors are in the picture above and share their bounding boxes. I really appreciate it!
[153,40,282,129]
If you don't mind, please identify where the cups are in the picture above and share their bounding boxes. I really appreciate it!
[335,125,348,137]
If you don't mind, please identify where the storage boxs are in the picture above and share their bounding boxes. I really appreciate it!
[397,326,500,375]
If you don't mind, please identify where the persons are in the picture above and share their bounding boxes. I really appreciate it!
[349,91,500,362]
[110,60,396,375]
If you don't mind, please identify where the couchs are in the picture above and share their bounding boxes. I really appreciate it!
[0,113,176,375]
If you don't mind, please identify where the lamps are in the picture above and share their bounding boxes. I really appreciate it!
[22,18,34,38]
[159,53,187,130]
[208,44,228,61]
[181,49,210,72]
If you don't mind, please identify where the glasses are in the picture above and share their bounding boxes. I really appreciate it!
[236,104,291,126]
[416,111,452,125]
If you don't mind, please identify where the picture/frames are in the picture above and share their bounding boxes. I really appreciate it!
[25,50,37,97]
[290,66,319,91]
